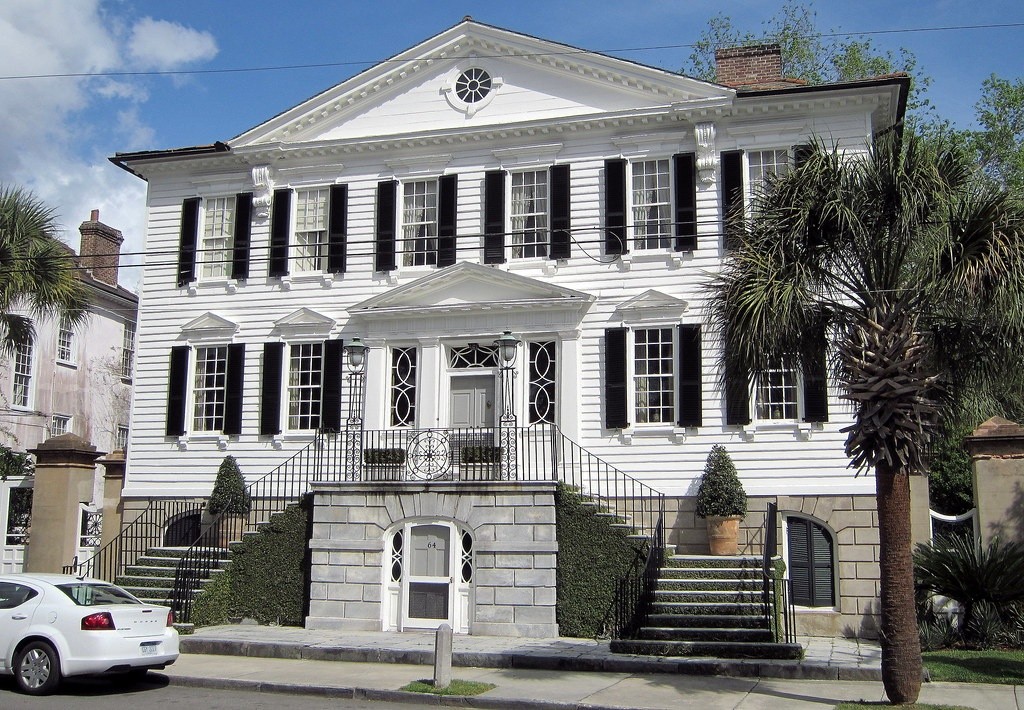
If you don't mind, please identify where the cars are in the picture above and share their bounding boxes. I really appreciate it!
[0,573,179,696]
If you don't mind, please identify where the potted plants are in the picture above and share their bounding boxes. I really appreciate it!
[211,453,252,552]
[459,447,506,481]
[361,448,407,481]
[693,440,750,555]
